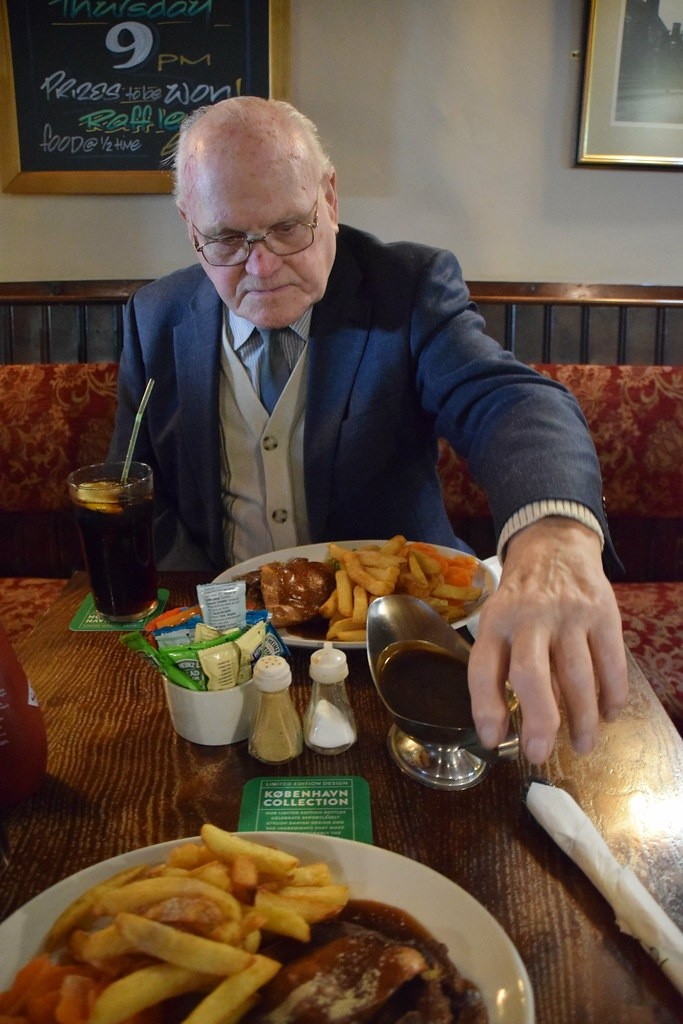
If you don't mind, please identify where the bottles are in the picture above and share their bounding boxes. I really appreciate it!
[248,656,302,763]
[304,642,357,755]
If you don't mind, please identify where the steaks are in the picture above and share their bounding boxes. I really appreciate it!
[228,558,335,628]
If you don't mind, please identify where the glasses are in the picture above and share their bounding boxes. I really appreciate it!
[190,183,321,267]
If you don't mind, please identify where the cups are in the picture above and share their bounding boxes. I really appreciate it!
[67,461,157,621]
[367,593,521,792]
[160,671,261,747]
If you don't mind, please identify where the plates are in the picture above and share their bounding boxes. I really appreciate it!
[211,540,498,650]
[466,553,503,642]
[0,831,537,1023]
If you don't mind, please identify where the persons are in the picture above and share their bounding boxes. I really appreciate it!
[102,94,630,767]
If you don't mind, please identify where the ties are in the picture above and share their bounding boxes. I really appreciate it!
[256,325,290,416]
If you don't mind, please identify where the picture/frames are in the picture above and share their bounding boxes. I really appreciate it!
[0,0,290,197]
[571,0,683,173]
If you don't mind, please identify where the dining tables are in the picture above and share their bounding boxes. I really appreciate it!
[0,576,683,1021]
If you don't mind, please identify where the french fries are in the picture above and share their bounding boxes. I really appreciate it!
[325,535,481,642]
[1,824,350,1024]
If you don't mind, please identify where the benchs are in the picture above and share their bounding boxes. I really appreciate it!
[1,364,683,736]
